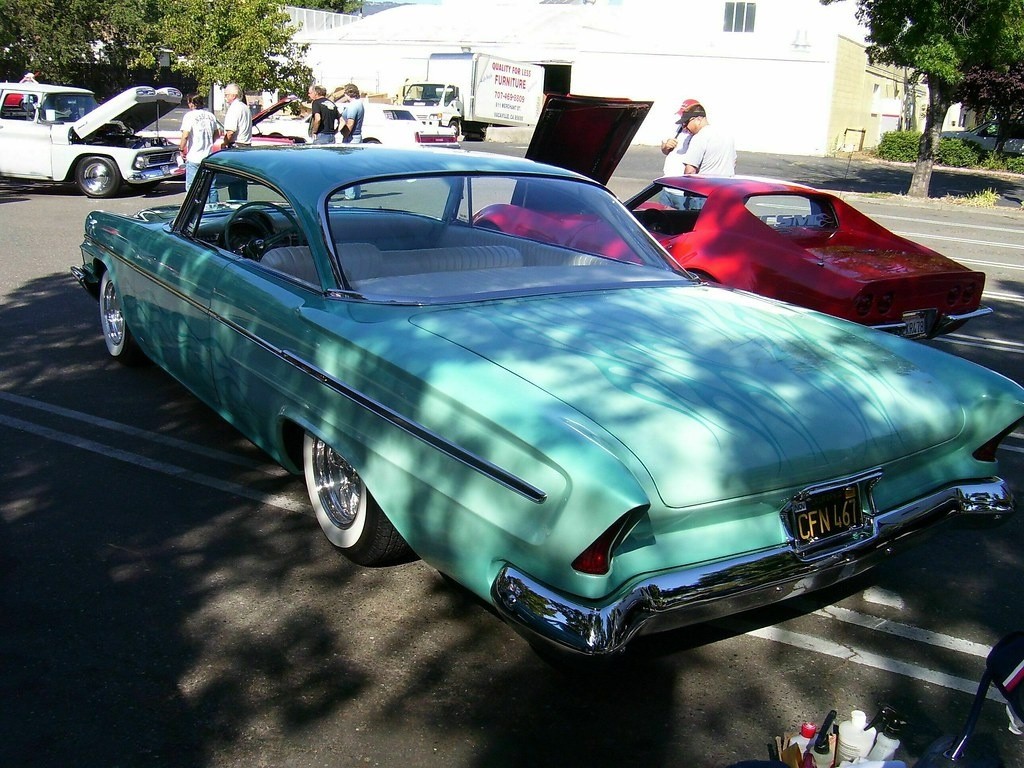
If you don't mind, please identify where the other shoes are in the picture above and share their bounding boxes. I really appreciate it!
[336,196,360,201]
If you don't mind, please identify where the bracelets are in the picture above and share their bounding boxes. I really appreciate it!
[178,148,184,152]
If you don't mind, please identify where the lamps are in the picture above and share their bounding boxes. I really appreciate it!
[460,46,470,54]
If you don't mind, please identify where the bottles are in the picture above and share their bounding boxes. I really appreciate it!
[835,710,877,766]
[788,721,819,764]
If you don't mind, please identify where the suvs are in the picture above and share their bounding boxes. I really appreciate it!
[939,119,1024,158]
[0,83,185,199]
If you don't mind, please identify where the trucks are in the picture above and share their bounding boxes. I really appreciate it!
[402,51,545,138]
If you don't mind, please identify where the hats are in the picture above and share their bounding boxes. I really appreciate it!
[676,98,700,117]
[675,104,706,124]
[24,73,35,77]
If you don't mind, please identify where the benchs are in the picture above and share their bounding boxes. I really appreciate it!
[261,242,523,286]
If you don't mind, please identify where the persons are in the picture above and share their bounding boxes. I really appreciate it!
[19,73,39,112]
[221,83,252,200]
[659,99,737,210]
[179,93,221,202]
[308,84,364,201]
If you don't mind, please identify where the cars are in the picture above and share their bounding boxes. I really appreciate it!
[471,93,994,338]
[157,94,305,177]
[71,144,1024,658]
[254,102,457,144]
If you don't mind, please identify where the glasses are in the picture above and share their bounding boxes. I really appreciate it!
[682,116,699,127]
